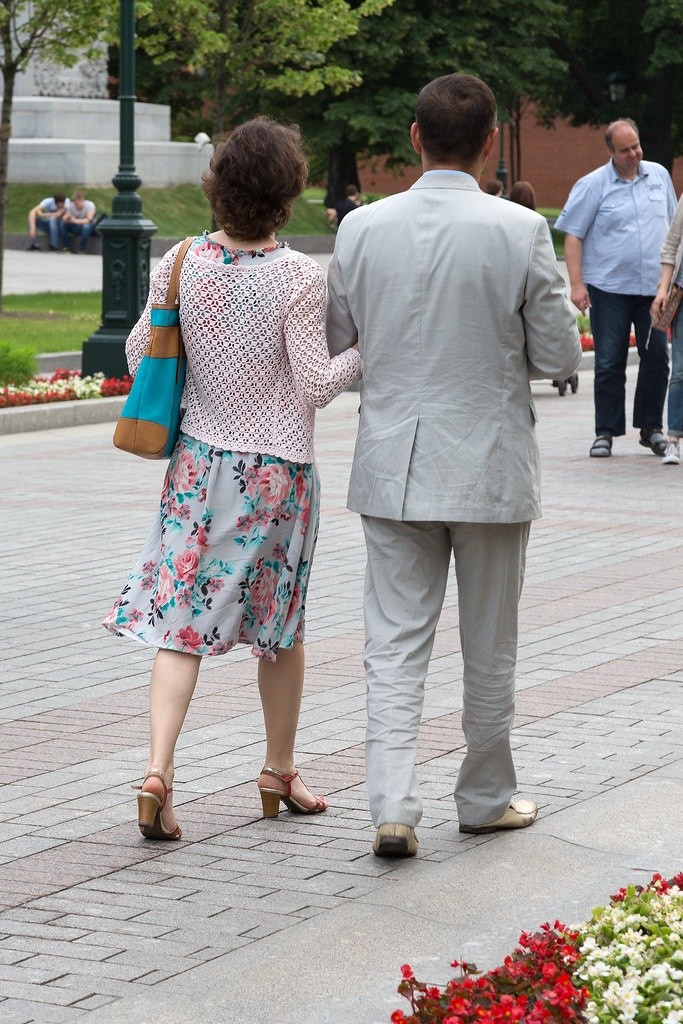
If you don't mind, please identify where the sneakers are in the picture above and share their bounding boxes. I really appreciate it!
[661,440,681,464]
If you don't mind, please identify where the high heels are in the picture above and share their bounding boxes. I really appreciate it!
[255,765,329,820]
[131,769,183,841]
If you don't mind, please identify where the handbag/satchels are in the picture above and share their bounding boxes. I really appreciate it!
[111,232,199,463]
[650,284,682,332]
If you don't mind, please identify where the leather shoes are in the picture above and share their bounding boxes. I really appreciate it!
[369,823,420,859]
[457,795,538,835]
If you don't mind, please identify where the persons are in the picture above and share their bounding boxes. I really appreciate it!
[26,193,69,252]
[485,179,503,197]
[554,118,677,459]
[61,190,97,253]
[327,74,583,858]
[104,118,364,839]
[649,192,683,465]
[509,181,535,211]
[336,185,357,227]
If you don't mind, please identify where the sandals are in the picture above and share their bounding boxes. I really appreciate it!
[589,434,612,457]
[638,427,667,456]
